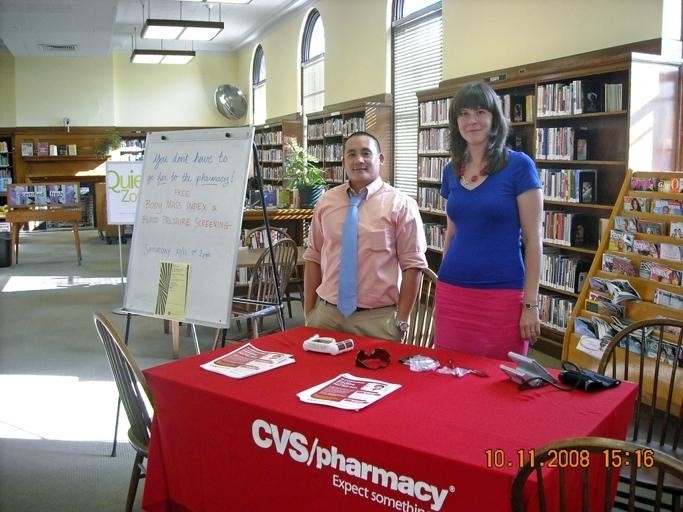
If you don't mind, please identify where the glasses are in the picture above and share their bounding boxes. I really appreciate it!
[355,347,392,371]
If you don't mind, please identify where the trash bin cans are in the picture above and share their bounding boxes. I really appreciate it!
[0,232,13,267]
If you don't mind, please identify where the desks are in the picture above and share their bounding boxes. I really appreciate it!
[4,207,82,267]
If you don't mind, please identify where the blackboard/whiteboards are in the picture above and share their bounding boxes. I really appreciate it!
[112,126,255,330]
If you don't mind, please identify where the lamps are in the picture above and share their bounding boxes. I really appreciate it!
[128,26,195,66]
[140,1,224,42]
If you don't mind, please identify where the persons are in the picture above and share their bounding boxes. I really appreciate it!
[301,131,429,344]
[431,79,546,361]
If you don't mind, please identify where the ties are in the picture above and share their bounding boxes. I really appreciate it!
[336,187,367,319]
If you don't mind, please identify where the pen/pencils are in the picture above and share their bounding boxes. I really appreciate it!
[448,359,459,376]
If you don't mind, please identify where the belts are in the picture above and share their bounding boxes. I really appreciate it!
[315,295,397,312]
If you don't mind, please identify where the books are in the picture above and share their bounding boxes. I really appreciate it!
[237,225,286,282]
[0,139,79,207]
[496,82,597,329]
[253,115,366,206]
[567,174,683,368]
[420,98,453,250]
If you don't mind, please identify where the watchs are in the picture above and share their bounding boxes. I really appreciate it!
[393,318,409,333]
